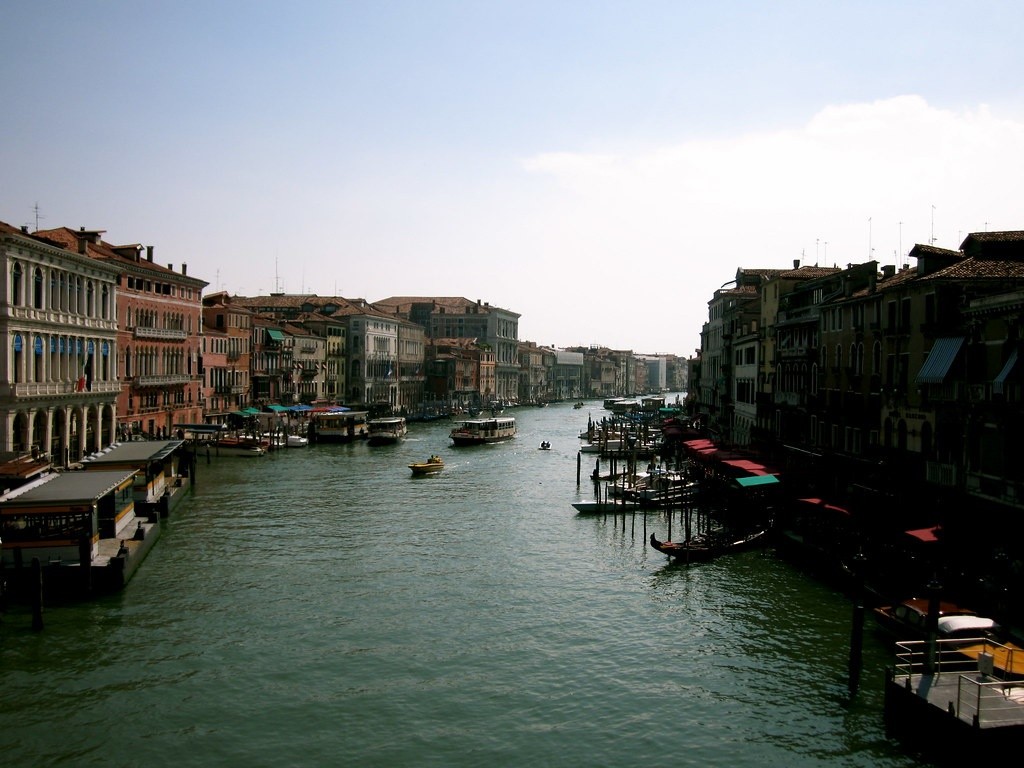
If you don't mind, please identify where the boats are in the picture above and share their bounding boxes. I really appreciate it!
[871,597,1024,681]
[449,418,516,445]
[612,402,640,414]
[408,459,445,473]
[574,404,582,408]
[571,413,699,512]
[172,433,309,456]
[538,440,552,450]
[603,398,626,409]
[367,417,408,443]
[640,398,665,411]
[650,523,768,559]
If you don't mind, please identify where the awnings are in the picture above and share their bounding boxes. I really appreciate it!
[265,403,288,414]
[268,329,286,341]
[230,407,260,418]
[796,496,849,516]
[286,405,315,412]
[325,405,351,412]
[902,525,945,544]
[734,474,781,487]
[662,418,780,478]
[307,407,328,412]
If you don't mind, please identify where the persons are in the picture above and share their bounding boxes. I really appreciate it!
[431,455,442,463]
